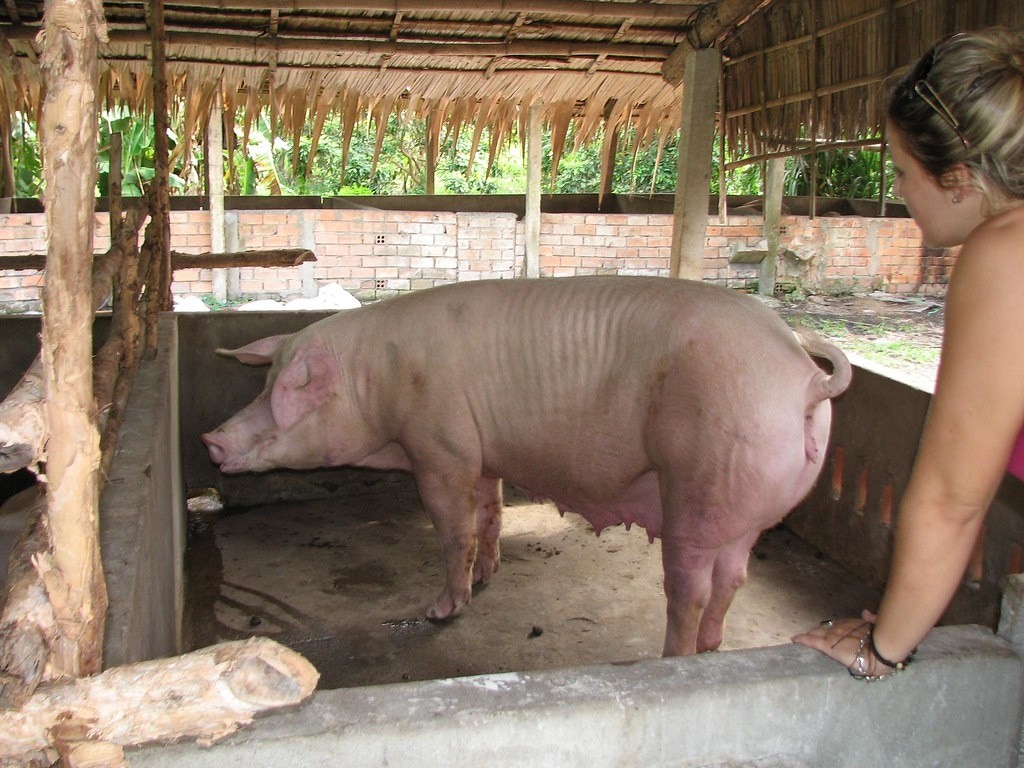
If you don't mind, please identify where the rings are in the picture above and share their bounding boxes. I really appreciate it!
[819,619,836,629]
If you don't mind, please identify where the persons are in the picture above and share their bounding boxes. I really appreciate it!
[791,26,1023,675]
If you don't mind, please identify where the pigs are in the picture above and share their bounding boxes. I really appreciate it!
[197,275,852,658]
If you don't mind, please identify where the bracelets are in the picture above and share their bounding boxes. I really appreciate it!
[843,621,919,680]
